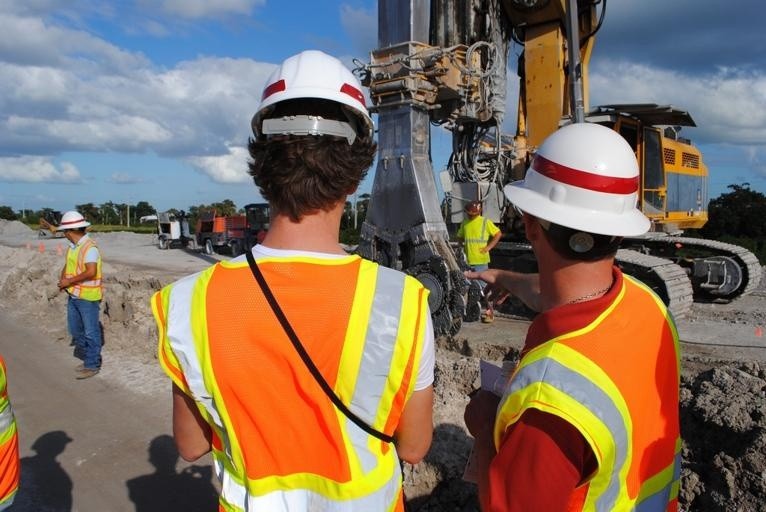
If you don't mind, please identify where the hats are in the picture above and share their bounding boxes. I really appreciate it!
[249,51,375,146]
[56,211,91,231]
[463,202,483,215]
[502,122,651,237]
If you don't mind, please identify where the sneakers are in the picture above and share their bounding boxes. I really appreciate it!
[482,311,496,323]
[75,362,99,379]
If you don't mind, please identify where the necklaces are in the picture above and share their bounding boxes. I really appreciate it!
[537,285,617,309]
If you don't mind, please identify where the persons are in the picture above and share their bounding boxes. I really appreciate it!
[452,200,506,324]
[1,343,28,512]
[460,113,682,512]
[148,50,435,512]
[42,209,120,383]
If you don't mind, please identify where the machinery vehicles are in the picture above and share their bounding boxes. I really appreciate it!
[196,206,271,254]
[349,1,761,334]
